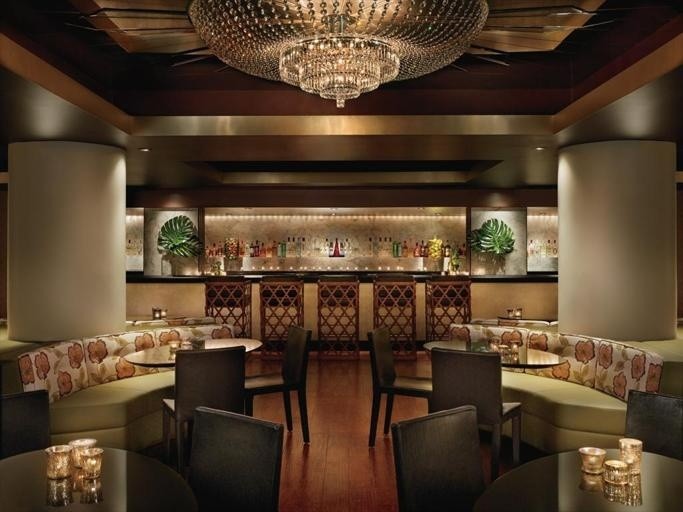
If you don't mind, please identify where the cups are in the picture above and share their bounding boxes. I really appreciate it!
[168,341,180,351]
[618,438,645,477]
[82,476,104,505]
[181,342,192,350]
[511,353,520,363]
[604,485,626,504]
[44,447,74,480]
[48,478,74,507]
[510,340,520,353]
[507,310,514,317]
[161,308,168,318]
[169,352,177,361]
[626,477,645,509]
[71,468,88,492]
[580,469,604,495]
[577,447,607,474]
[604,459,630,486]
[153,308,160,319]
[502,355,509,362]
[498,344,509,354]
[516,307,522,316]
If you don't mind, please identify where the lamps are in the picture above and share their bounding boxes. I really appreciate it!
[603,481,627,503]
[181,2,491,110]
[511,344,520,360]
[515,307,522,318]
[506,308,514,319]
[152,307,167,321]
[619,438,643,475]
[80,478,103,505]
[43,445,72,481]
[71,466,82,491]
[578,446,604,473]
[498,344,510,360]
[490,343,499,355]
[577,472,604,493]
[625,473,642,506]
[603,460,628,483]
[45,477,73,506]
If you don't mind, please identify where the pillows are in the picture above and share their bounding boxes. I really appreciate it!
[319,274,358,306]
[205,276,244,308]
[261,275,301,307]
[377,275,415,306]
[431,274,469,307]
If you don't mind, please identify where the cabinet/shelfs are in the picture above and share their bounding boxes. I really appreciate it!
[198,232,464,270]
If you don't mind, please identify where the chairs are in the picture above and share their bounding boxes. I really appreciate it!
[366,326,436,447]
[243,325,312,446]
[261,281,307,359]
[179,408,284,511]
[0,389,51,462]
[371,280,419,361]
[316,282,361,358]
[624,389,683,463]
[160,344,246,466]
[390,404,486,511]
[425,281,471,344]
[429,347,522,479]
[204,282,251,338]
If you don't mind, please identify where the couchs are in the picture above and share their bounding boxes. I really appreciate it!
[447,323,664,452]
[18,325,234,453]
